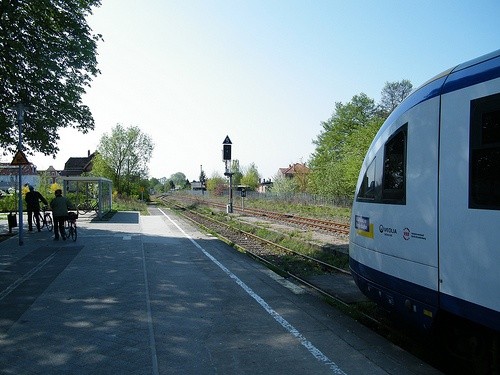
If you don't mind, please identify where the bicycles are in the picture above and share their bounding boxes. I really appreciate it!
[64,208,78,242]
[33,200,53,232]
[76,196,99,215]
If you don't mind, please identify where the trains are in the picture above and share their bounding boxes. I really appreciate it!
[348,49,500,333]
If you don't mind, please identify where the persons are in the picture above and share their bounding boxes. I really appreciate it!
[50,189,74,240]
[25,186,48,232]
